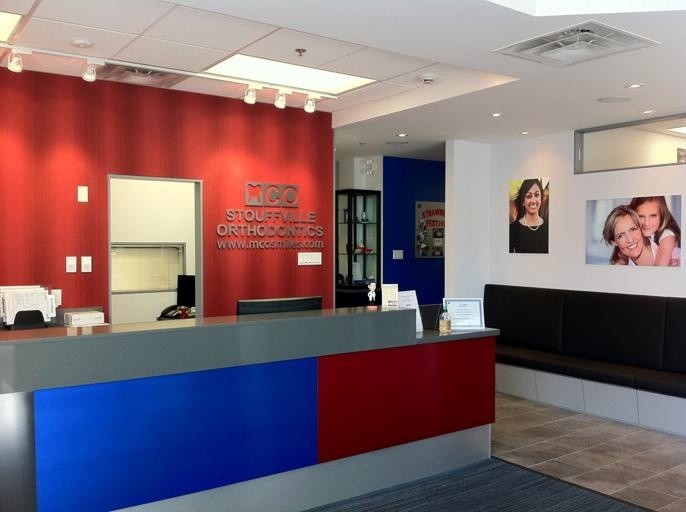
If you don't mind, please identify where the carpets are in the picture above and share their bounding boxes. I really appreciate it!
[304,454,658,510]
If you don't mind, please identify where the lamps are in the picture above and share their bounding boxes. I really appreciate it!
[5,52,97,84]
[243,83,322,114]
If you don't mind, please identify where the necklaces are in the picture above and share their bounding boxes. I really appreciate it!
[524,213,541,232]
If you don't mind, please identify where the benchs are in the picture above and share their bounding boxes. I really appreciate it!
[483,283,686,438]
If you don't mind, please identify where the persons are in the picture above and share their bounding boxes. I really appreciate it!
[367,281,379,311]
[610,195,681,265]
[602,204,680,266]
[509,178,548,253]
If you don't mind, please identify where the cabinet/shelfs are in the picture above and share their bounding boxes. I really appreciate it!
[335,188,383,307]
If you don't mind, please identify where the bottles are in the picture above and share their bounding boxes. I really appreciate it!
[439,308,452,333]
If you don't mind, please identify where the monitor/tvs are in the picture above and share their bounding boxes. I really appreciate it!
[177,273,195,308]
[237,295,324,315]
